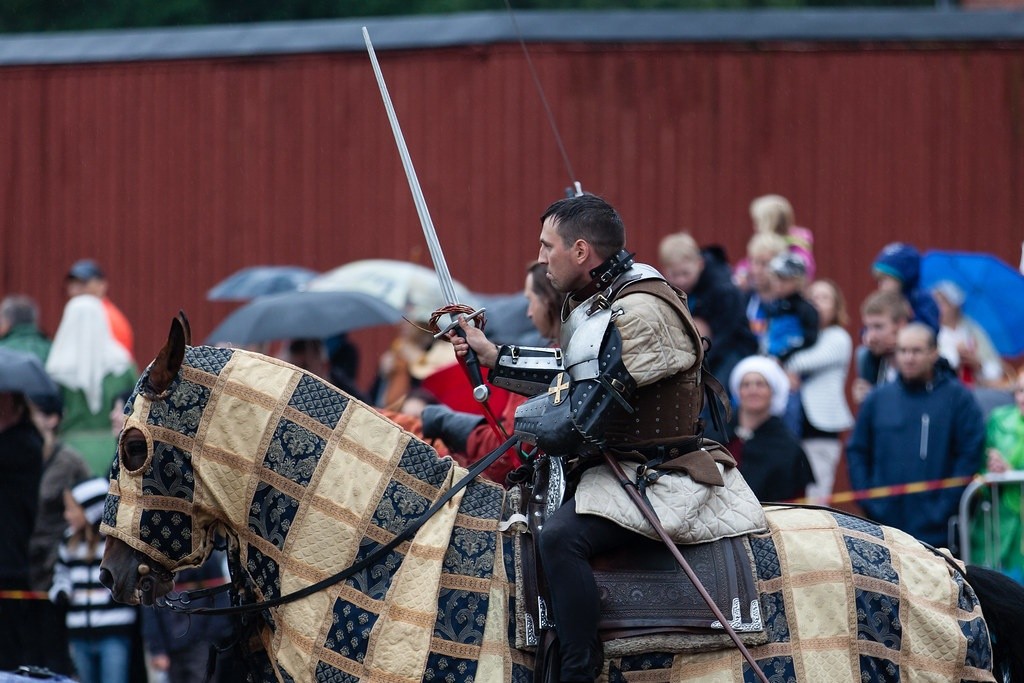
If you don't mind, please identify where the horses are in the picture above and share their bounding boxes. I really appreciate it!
[99,308,1024,683]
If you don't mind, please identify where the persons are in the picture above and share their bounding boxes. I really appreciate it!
[266,306,459,455]
[440,192,706,683]
[640,193,1024,622]
[418,250,580,507]
[1,248,225,683]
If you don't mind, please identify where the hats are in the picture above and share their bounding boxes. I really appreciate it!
[64,257,104,285]
[768,252,806,281]
[728,354,791,416]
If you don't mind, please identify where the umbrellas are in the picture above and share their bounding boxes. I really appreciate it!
[207,262,320,301]
[298,255,485,334]
[205,283,409,346]
